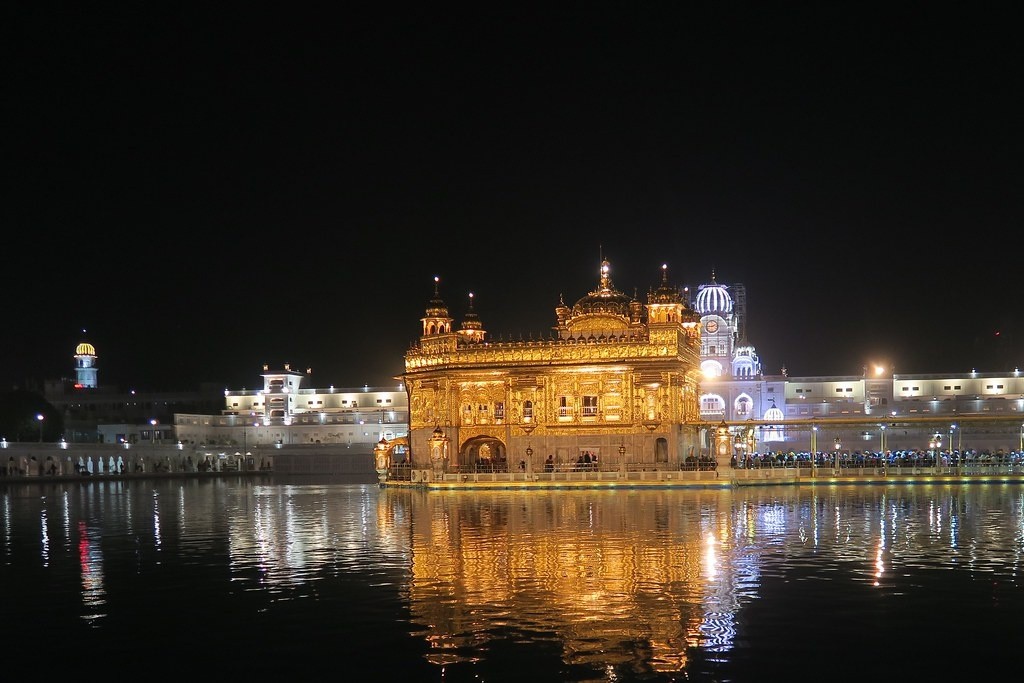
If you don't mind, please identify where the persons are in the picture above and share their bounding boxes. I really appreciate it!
[680,455,715,471]
[120,462,124,474]
[177,453,216,470]
[815,452,882,468]
[885,449,959,467]
[480,457,506,473]
[545,455,554,472]
[134,454,143,472]
[518,459,525,468]
[961,449,1024,466]
[572,451,597,472]
[732,451,812,468]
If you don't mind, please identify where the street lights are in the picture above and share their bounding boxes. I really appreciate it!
[151,420,156,443]
[834,438,841,468]
[936,435,941,468]
[38,415,43,442]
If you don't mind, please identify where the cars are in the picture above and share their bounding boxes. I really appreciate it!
[218,454,225,460]
